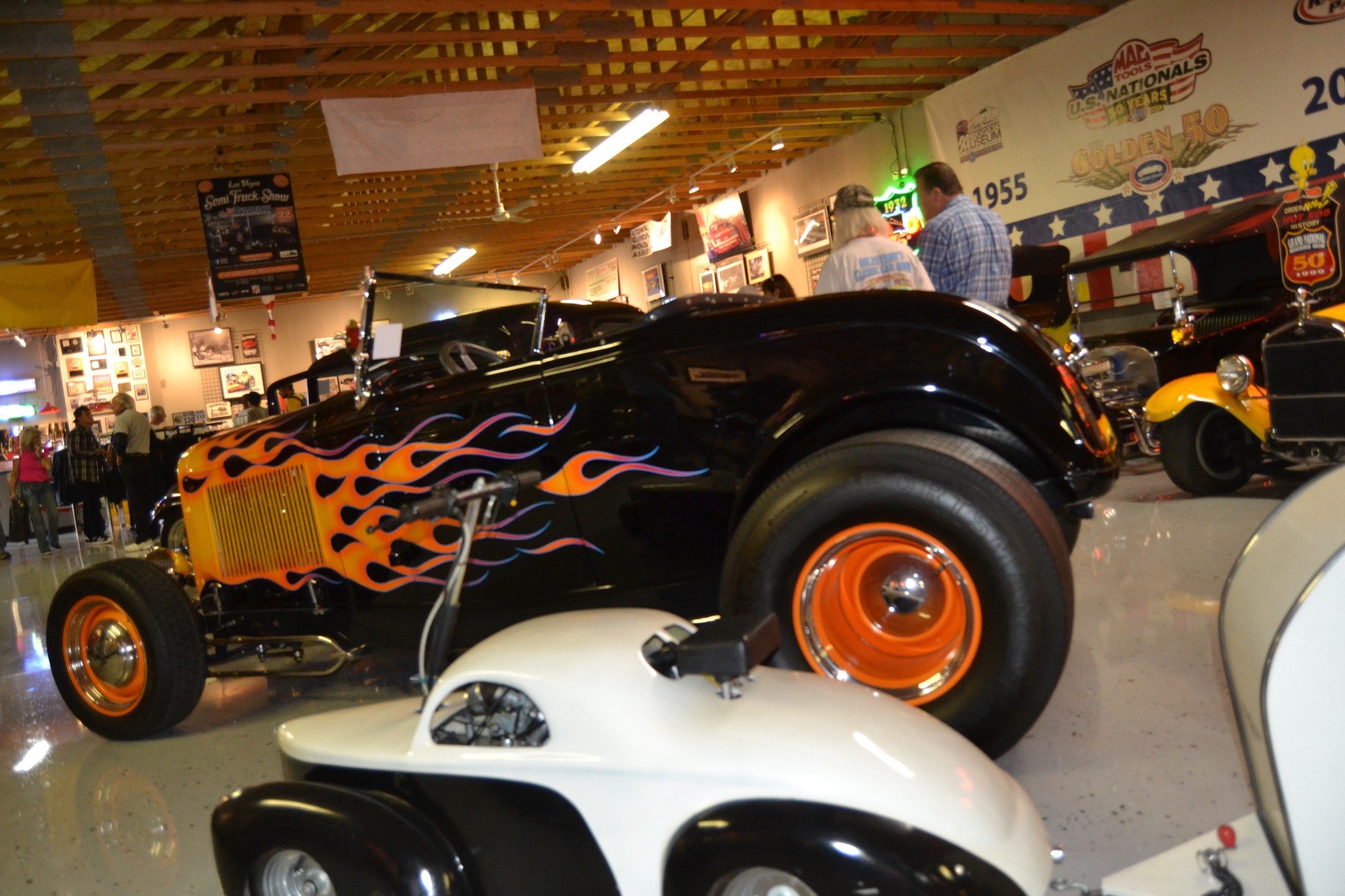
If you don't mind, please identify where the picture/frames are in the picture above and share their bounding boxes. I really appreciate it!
[240,333,260,359]
[792,203,833,260]
[315,336,347,361]
[641,262,666,303]
[132,369,146,380]
[716,260,747,294]
[105,417,116,434]
[745,248,772,286]
[130,344,141,357]
[188,327,236,369]
[134,383,148,401]
[698,269,717,294]
[217,361,266,401]
[110,329,123,344]
[93,373,113,396]
[118,382,132,392]
[338,375,357,393]
[125,326,140,343]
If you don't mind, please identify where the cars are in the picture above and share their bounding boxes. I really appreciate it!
[1061,178,1345,460]
[47,268,1123,764]
[1142,288,1343,499]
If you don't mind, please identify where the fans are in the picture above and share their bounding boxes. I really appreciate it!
[439,164,537,225]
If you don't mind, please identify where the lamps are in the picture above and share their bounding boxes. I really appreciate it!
[117,323,126,334]
[433,246,477,277]
[36,335,60,415]
[14,329,31,348]
[89,329,97,339]
[162,320,170,328]
[219,309,227,321]
[572,108,670,174]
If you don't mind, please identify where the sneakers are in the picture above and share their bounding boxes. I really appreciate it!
[0,549,11,560]
[42,549,52,555]
[51,543,63,551]
[87,534,113,545]
[124,537,162,552]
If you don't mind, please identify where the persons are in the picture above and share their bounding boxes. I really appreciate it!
[149,382,310,441]
[762,273,797,302]
[814,183,936,295]
[0,520,11,560]
[110,393,160,552]
[9,426,62,555]
[67,406,114,545]
[914,161,1013,312]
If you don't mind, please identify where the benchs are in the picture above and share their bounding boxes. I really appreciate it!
[1184,244,1287,330]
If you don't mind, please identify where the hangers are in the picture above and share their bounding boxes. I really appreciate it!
[154,422,235,439]
[56,433,114,452]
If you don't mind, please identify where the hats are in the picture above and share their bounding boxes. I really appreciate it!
[72,406,89,423]
[828,183,876,222]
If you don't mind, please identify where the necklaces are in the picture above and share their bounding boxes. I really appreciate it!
[25,448,39,474]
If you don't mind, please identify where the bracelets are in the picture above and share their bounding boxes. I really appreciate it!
[37,455,44,461]
